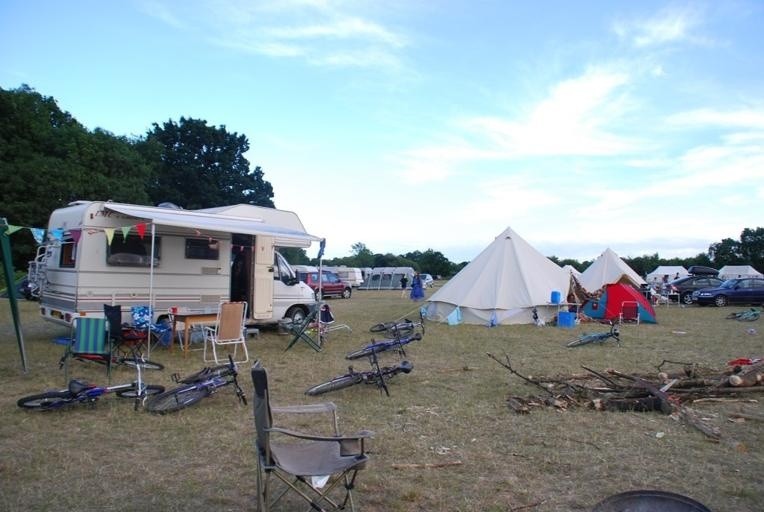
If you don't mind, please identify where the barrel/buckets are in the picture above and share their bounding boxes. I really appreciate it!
[278,321,288,335]
[551,291,560,303]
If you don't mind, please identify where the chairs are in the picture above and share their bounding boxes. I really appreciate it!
[63,301,248,390]
[619,301,641,327]
[250,360,375,512]
[279,301,325,354]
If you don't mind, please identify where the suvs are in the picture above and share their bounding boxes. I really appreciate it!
[419,273,433,289]
[302,269,352,300]
[691,277,764,307]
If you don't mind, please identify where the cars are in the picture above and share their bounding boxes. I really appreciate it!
[18,279,39,299]
[661,274,724,306]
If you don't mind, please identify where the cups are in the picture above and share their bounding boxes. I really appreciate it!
[168,306,178,313]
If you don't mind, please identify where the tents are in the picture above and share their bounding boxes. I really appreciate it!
[717,265,763,280]
[645,265,690,286]
[423,227,571,325]
[579,247,661,306]
[562,265,580,283]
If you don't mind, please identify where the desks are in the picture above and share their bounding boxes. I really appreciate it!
[640,291,680,308]
[536,303,582,328]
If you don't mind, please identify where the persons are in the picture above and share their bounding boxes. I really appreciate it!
[409,271,424,302]
[399,275,408,299]
[652,276,658,302]
[661,274,676,295]
[674,272,679,279]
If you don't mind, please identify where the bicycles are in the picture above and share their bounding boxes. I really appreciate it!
[147,354,247,414]
[568,319,619,347]
[307,345,413,396]
[16,365,165,411]
[347,310,425,360]
[57,337,163,371]
[728,306,760,322]
